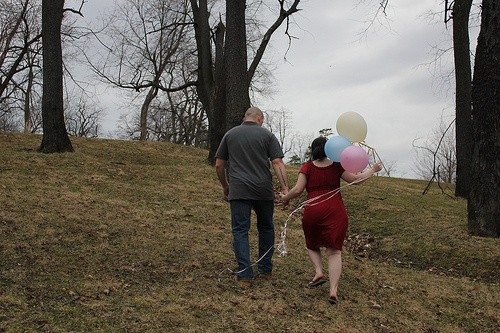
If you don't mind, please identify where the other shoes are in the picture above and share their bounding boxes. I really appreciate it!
[236,265,273,281]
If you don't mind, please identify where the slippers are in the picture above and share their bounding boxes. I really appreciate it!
[309,277,339,304]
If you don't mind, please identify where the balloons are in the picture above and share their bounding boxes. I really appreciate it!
[340,145,370,173]
[336,111,368,143]
[323,136,353,163]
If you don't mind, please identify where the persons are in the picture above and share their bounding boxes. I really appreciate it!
[214,105,290,287]
[278,136,383,304]
